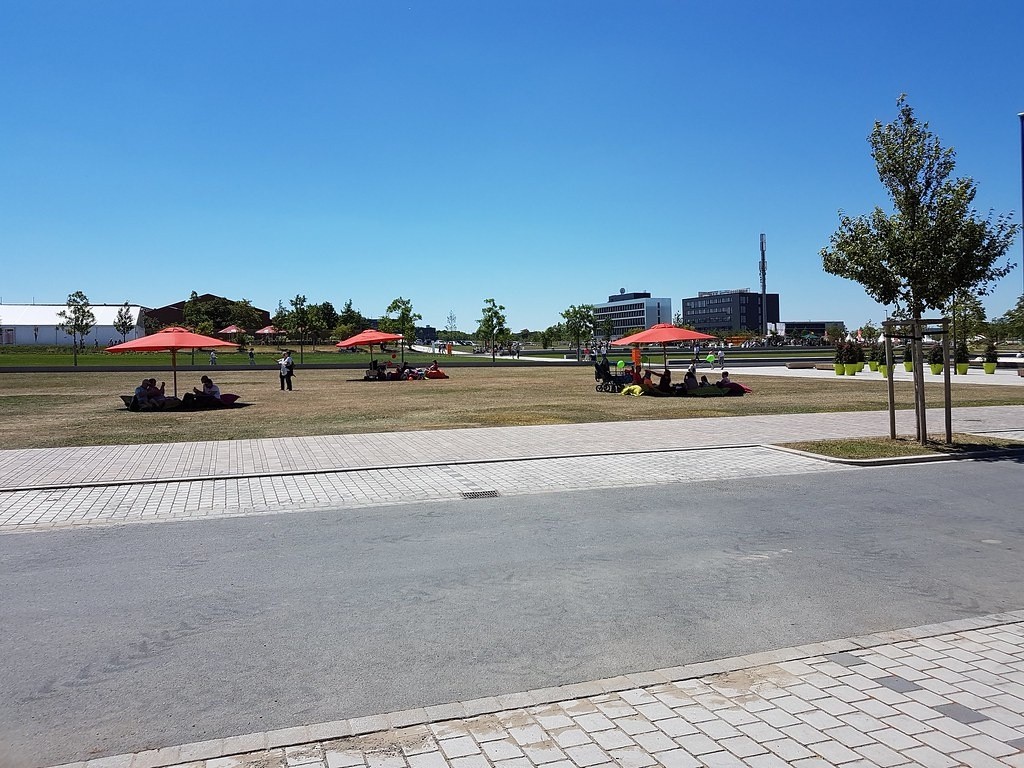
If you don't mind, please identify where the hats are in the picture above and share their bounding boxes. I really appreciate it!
[142,379,151,385]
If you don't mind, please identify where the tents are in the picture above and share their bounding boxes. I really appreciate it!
[845,333,935,344]
[802,334,817,346]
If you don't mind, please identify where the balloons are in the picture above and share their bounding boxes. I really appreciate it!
[617,360,624,368]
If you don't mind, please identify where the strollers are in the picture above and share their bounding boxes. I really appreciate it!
[594,360,625,393]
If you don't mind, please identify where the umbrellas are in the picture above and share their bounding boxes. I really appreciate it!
[337,329,406,370]
[611,323,719,370]
[218,324,285,339]
[105,325,241,395]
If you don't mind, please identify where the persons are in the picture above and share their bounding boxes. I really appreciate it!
[186,375,220,411]
[684,359,710,390]
[482,339,521,359]
[261,335,266,345]
[74,338,123,355]
[127,378,165,411]
[631,366,671,395]
[704,351,715,363]
[716,372,730,389]
[395,359,438,381]
[569,339,611,361]
[696,351,700,359]
[248,348,256,365]
[380,341,387,350]
[439,342,452,354]
[632,344,641,369]
[210,350,217,365]
[718,348,724,370]
[272,350,295,392]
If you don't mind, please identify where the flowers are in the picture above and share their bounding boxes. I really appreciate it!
[832,340,866,364]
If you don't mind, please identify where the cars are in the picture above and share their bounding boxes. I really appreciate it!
[512,340,535,346]
[413,338,479,347]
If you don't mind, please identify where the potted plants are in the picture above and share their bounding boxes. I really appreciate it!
[901,344,913,372]
[954,342,970,374]
[926,344,944,375]
[981,342,998,374]
[868,340,897,377]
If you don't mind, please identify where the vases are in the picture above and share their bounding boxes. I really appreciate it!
[833,362,865,376]
